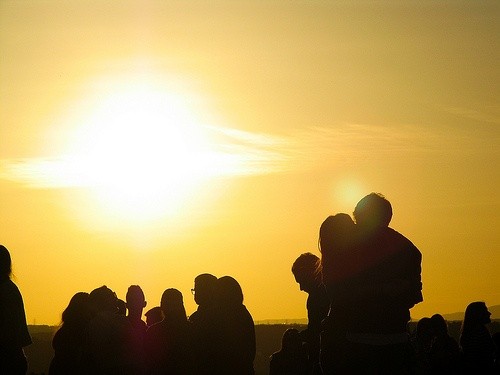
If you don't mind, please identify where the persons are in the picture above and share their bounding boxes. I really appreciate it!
[47,272,258,375]
[267,192,500,375]
[0,244,33,375]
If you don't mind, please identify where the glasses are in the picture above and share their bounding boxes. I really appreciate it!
[191,288,195,294]
